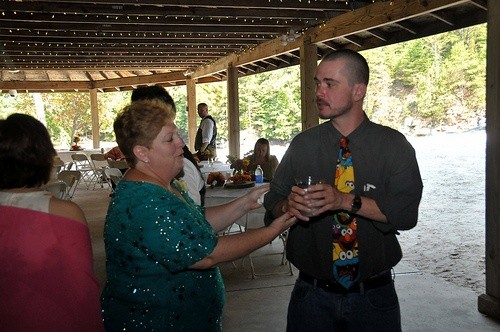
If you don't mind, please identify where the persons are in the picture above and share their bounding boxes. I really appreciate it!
[245,138,279,183]
[131,85,206,207]
[103,145,130,197]
[102,99,298,332]
[264,50,423,332]
[0,113,104,332]
[193,103,217,161]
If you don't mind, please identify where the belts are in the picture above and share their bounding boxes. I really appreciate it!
[298,270,392,292]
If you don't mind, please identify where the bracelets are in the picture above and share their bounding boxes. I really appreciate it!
[198,151,203,155]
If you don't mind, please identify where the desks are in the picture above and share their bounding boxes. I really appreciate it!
[203,179,271,269]
[200,167,230,177]
[56,149,106,171]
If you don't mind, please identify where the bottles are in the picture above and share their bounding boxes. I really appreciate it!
[255,165,264,185]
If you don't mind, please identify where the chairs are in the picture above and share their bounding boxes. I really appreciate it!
[45,149,128,201]
[240,212,295,278]
[198,161,233,183]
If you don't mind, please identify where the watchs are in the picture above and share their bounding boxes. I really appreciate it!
[348,193,362,215]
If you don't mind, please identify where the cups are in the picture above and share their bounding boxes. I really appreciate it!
[293,176,327,217]
[101,148,104,153]
[208,158,213,166]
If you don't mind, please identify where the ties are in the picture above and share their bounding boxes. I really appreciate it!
[331,138,360,289]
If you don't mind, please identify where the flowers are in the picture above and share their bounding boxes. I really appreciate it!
[225,150,258,176]
[207,170,225,189]
[204,146,218,162]
[73,136,80,146]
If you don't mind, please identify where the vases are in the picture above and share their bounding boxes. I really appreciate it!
[72,145,80,150]
[229,176,251,183]
[208,157,214,166]
